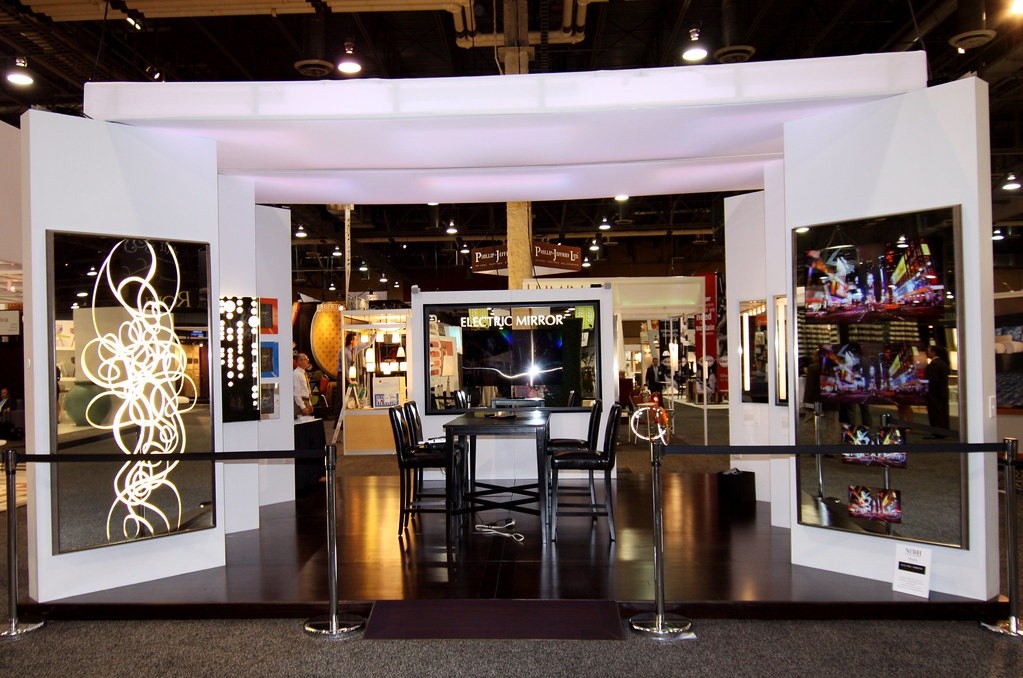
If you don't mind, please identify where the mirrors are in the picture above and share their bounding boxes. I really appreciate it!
[219,296,262,423]
[45,231,217,557]
[422,299,603,416]
[786,202,974,553]
[772,294,790,406]
[738,299,770,404]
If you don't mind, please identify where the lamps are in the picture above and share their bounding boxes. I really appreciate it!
[379,273,388,283]
[441,355,458,397]
[334,36,362,78]
[4,57,38,88]
[742,312,752,392]
[1001,174,1022,191]
[460,242,470,254]
[581,256,591,268]
[680,25,710,64]
[589,238,600,251]
[126,9,143,31]
[393,281,399,288]
[777,297,787,400]
[598,217,611,230]
[446,219,458,235]
[295,224,308,238]
[349,315,408,378]
[669,337,679,372]
[331,244,343,257]
[992,230,1004,241]
[328,283,337,291]
[144,64,160,81]
[359,261,369,272]
[614,196,629,202]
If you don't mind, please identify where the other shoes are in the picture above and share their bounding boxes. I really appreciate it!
[923,433,949,439]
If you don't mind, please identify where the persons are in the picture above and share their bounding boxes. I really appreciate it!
[0,388,16,417]
[708,368,716,392]
[798,349,825,417]
[923,346,950,439]
[293,352,313,419]
[837,348,856,440]
[646,358,667,408]
[333,332,377,429]
[892,345,915,434]
[679,356,689,376]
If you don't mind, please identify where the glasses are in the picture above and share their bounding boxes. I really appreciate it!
[300,358,309,362]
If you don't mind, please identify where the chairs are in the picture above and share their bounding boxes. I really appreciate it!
[404,400,470,518]
[387,405,462,538]
[453,389,464,409]
[460,390,470,408]
[621,410,633,442]
[567,390,575,407]
[547,399,602,515]
[549,402,623,542]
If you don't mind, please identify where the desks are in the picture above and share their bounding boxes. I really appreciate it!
[634,402,658,446]
[491,397,546,407]
[442,407,553,548]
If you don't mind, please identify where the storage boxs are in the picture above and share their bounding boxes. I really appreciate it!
[581,399,596,407]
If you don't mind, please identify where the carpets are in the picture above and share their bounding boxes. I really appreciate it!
[363,598,630,642]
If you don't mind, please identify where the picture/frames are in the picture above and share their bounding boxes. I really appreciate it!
[260,298,279,335]
[261,341,280,378]
[431,335,458,380]
[261,383,280,419]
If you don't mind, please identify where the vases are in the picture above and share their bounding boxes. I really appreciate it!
[62,378,111,429]
